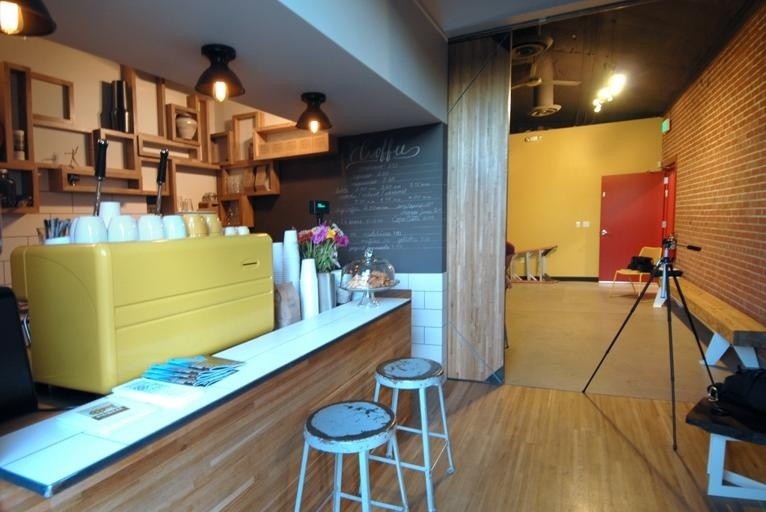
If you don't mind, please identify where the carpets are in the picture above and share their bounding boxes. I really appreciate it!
[505,273,731,403]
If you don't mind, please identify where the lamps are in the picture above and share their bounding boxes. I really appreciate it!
[0,1,56,36]
[295,91,333,134]
[591,14,626,114]
[196,42,244,103]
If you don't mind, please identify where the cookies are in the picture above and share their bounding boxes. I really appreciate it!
[346,271,396,289]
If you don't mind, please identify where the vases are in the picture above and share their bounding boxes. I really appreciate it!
[318,271,336,311]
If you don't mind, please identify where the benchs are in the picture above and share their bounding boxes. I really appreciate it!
[653,274,766,373]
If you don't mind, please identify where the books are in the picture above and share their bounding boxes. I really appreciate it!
[141,354,245,388]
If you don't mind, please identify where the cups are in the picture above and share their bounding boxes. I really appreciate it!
[317,273,336,315]
[271,228,318,324]
[223,225,249,236]
[42,235,73,244]
[96,200,120,216]
[183,199,194,212]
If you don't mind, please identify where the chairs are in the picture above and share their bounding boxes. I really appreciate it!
[609,246,664,303]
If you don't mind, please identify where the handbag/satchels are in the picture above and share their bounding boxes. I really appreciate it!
[706,364,766,434]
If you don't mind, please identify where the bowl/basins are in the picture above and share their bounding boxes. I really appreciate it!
[66,214,222,243]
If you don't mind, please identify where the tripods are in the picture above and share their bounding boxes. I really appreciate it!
[582,260,715,450]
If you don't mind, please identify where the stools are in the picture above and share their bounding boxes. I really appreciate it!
[359,357,456,511]
[294,401,410,511]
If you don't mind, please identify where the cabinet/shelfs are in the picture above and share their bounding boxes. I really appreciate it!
[1,57,330,227]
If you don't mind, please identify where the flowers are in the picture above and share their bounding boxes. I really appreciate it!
[297,219,349,270]
